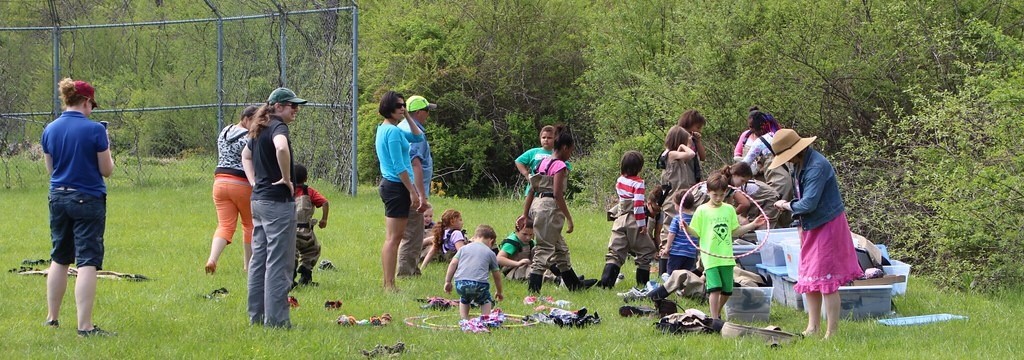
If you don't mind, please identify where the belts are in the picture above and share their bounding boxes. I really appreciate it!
[54,186,77,191]
[535,192,554,197]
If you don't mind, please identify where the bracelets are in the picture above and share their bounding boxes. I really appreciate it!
[781,201,787,209]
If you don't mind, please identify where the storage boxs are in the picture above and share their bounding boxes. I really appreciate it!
[724,226,912,322]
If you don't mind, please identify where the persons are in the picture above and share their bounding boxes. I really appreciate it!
[768,128,862,341]
[515,125,572,221]
[41,78,118,338]
[375,91,424,296]
[396,95,438,279]
[516,123,598,295]
[205,105,259,274]
[496,214,585,288]
[433,209,498,265]
[594,150,655,291]
[420,201,439,269]
[241,87,308,330]
[443,225,504,323]
[679,173,769,320]
[288,162,329,293]
[641,106,801,292]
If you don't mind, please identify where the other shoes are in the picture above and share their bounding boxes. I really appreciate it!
[288,296,298,308]
[521,263,722,333]
[205,261,216,275]
[318,261,337,272]
[325,300,342,310]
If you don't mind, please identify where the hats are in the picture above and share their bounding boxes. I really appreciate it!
[405,95,437,112]
[264,87,308,105]
[767,128,817,170]
[72,81,99,108]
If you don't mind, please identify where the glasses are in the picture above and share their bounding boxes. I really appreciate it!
[280,103,299,109]
[395,102,407,109]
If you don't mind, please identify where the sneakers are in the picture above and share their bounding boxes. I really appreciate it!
[42,319,60,329]
[76,324,118,340]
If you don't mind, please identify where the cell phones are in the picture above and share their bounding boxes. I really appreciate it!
[100,121,107,128]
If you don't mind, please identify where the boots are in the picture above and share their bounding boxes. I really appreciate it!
[299,271,320,288]
[290,272,299,289]
